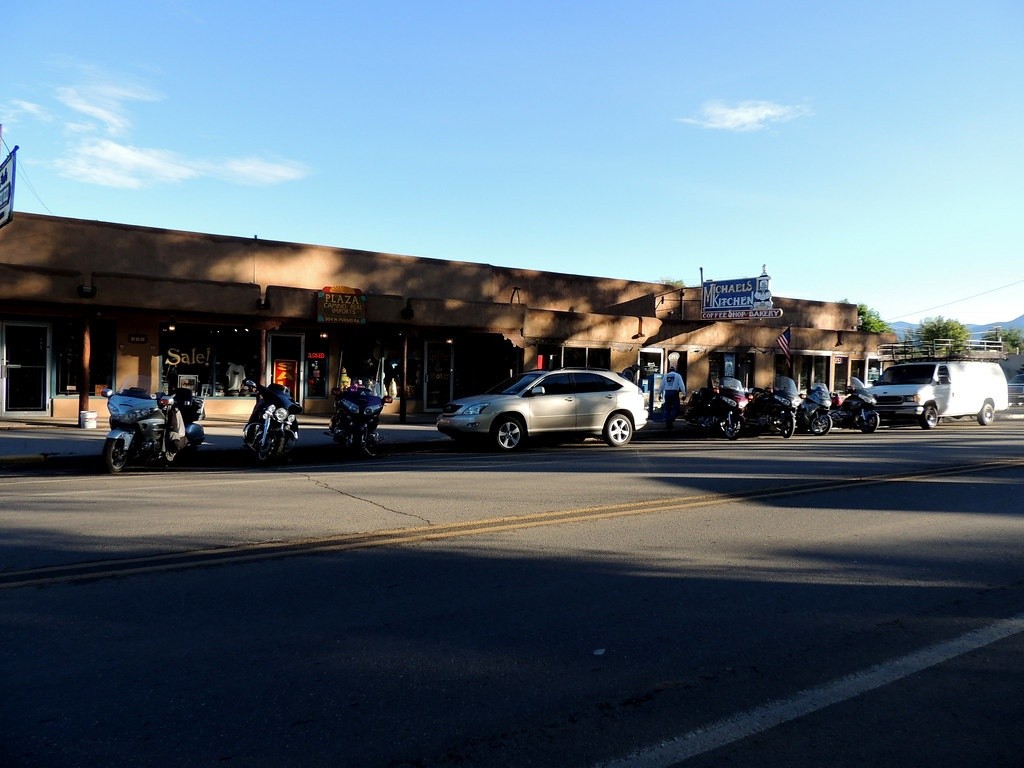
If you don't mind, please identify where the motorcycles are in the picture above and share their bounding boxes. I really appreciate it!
[829,376,881,434]
[685,376,748,440]
[101,373,206,474]
[795,380,834,437]
[239,378,302,466]
[744,376,800,439]
[323,349,392,461]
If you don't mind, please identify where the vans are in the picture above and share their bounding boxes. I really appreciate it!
[868,362,1010,429]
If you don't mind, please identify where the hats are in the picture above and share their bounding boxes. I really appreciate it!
[670,366,676,371]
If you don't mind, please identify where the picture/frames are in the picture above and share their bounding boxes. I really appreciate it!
[177,374,198,395]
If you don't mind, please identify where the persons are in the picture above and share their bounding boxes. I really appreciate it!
[622,363,641,383]
[658,366,686,429]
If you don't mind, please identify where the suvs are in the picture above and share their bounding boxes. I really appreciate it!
[1008,373,1024,405]
[437,367,648,451]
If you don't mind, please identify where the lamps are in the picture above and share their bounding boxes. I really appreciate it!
[400,308,414,319]
[256,297,271,309]
[78,282,97,298]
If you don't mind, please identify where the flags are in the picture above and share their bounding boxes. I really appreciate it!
[775,326,792,369]
